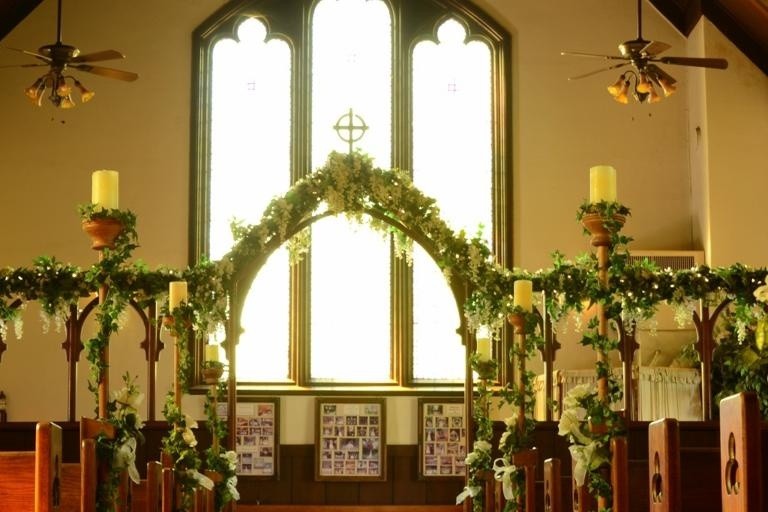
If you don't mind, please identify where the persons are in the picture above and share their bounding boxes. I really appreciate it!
[450,429,460,442]
[425,417,433,427]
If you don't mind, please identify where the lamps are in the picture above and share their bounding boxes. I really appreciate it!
[607,62,678,105]
[23,64,96,113]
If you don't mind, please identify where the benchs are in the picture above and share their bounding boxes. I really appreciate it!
[542,458,573,509]
[1,418,223,511]
[609,436,648,510]
[574,455,608,510]
[719,392,766,511]
[511,446,543,509]
[648,416,719,511]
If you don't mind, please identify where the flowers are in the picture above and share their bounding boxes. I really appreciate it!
[467,438,492,476]
[491,455,521,506]
[560,392,608,494]
[498,413,521,455]
[466,483,481,500]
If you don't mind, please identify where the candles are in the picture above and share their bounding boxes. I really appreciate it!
[204,343,218,362]
[168,277,190,313]
[512,278,532,314]
[90,168,119,212]
[477,337,491,363]
[589,163,616,203]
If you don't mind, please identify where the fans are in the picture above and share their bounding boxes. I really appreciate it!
[0,0,140,112]
[557,1,728,106]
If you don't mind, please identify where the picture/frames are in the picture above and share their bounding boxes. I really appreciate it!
[206,395,488,484]
